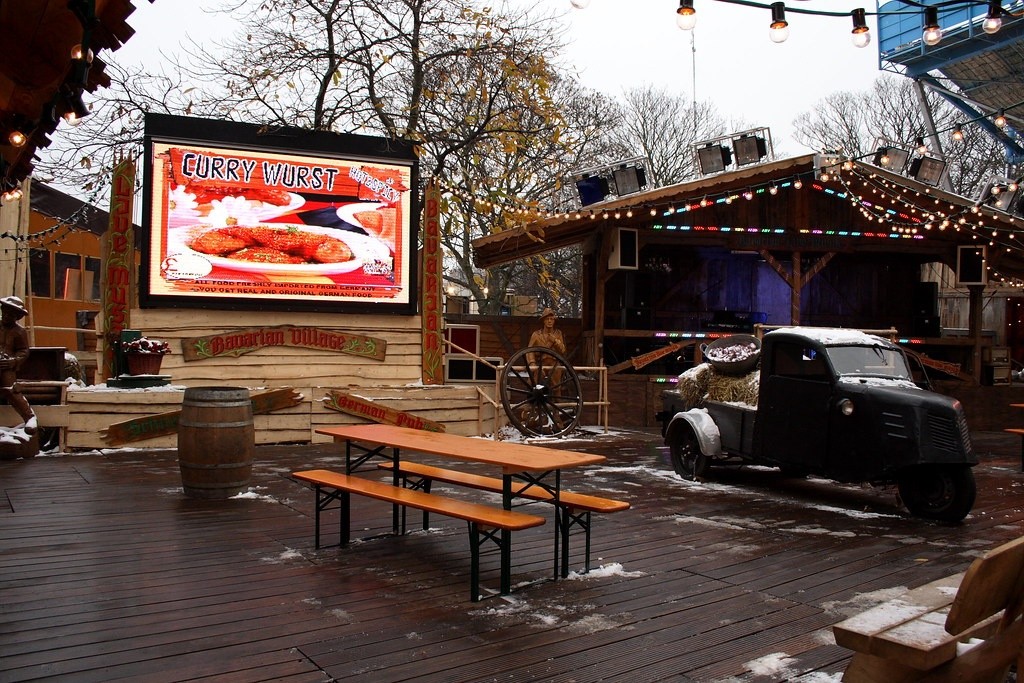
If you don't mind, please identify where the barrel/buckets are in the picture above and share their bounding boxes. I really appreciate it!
[178,386,255,506]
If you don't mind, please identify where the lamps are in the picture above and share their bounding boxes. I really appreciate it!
[697,143,731,173]
[576,173,610,207]
[612,165,645,197]
[912,156,946,187]
[733,135,766,166]
[981,175,1018,212]
[876,146,909,174]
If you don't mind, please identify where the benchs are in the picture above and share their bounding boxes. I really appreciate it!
[831,536,1024,683]
[292,469,546,602]
[376,462,630,579]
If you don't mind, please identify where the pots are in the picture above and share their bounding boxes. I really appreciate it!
[700,335,761,373]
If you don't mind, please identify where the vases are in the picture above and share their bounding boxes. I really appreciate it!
[124,352,163,375]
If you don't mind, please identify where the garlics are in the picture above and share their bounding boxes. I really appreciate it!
[708,342,760,362]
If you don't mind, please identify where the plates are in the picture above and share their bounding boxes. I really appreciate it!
[167,191,306,222]
[336,202,386,227]
[184,221,390,277]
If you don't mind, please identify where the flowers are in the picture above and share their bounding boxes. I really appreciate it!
[112,338,172,356]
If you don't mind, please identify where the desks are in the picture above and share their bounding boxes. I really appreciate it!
[313,423,576,594]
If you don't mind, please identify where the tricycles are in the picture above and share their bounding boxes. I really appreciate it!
[662,327,979,521]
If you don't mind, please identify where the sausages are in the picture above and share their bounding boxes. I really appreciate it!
[192,227,352,264]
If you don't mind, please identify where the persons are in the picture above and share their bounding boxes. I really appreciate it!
[526,308,566,429]
[0,297,38,424]
